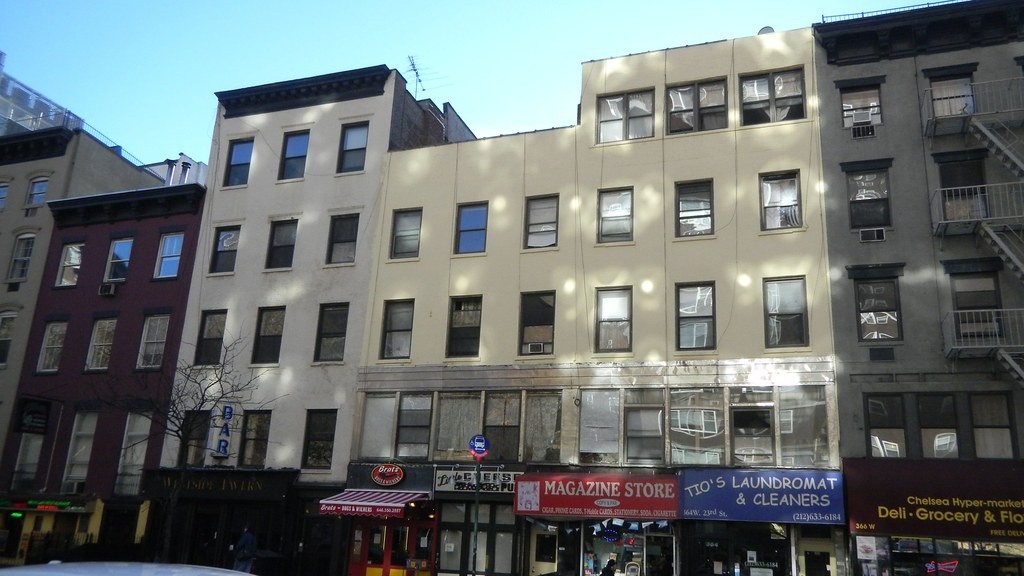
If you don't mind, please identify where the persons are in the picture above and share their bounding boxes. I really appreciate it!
[232,526,256,573]
[587,551,597,574]
[600,559,617,576]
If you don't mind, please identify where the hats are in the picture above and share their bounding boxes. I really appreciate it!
[607,560,617,566]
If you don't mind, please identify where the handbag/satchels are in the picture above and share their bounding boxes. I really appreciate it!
[238,548,252,561]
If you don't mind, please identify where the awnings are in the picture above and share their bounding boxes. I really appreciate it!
[318,489,430,519]
